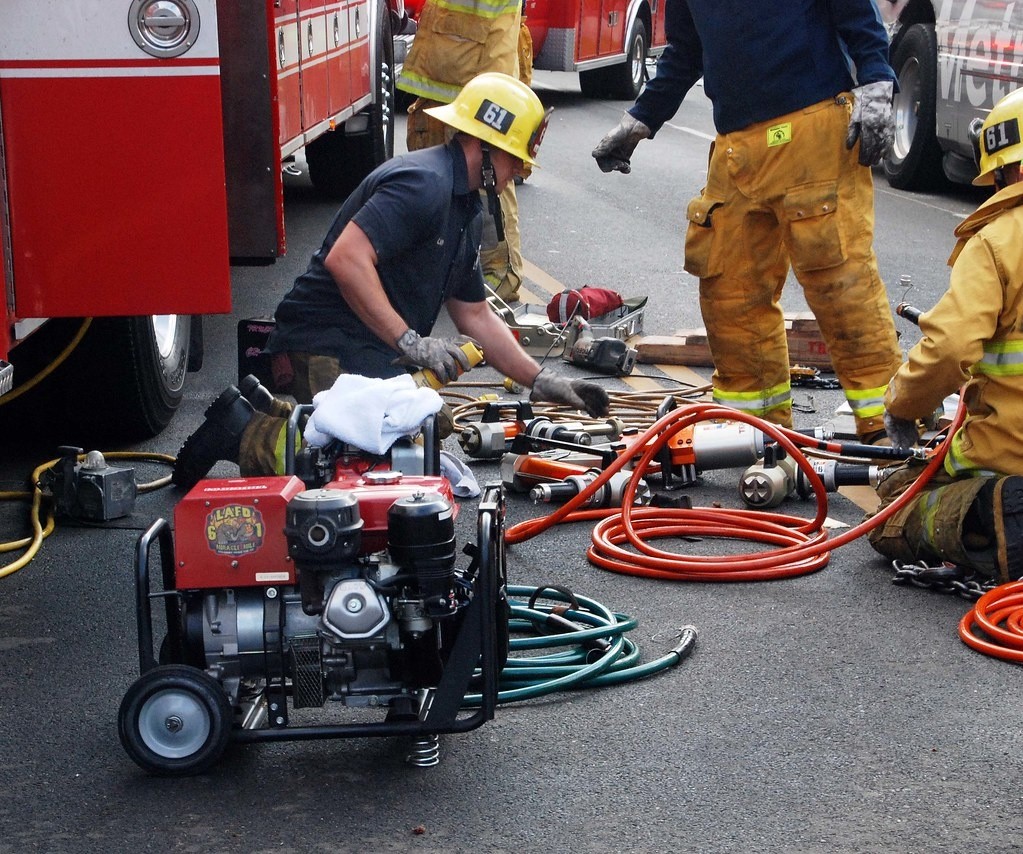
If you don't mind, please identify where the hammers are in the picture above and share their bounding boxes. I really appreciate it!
[447,375,526,394]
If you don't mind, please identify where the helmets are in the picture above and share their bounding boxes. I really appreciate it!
[972,86,1023,185]
[421,71,550,166]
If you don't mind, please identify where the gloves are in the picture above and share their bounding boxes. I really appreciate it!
[396,329,471,383]
[591,110,653,174]
[846,78,892,168]
[531,368,611,418]
[884,413,919,455]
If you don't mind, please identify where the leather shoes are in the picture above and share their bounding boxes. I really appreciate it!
[240,375,272,410]
[173,387,255,492]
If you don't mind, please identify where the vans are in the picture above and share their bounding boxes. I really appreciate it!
[875,0,1023,200]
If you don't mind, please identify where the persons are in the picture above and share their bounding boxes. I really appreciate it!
[171,72,609,492]
[862,87,1023,582]
[396,0,534,309]
[591,0,918,470]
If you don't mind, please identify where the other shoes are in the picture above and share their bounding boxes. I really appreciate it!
[861,432,889,446]
[975,474,1023,581]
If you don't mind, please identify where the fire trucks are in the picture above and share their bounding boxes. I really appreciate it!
[379,0,673,107]
[0,0,396,441]
